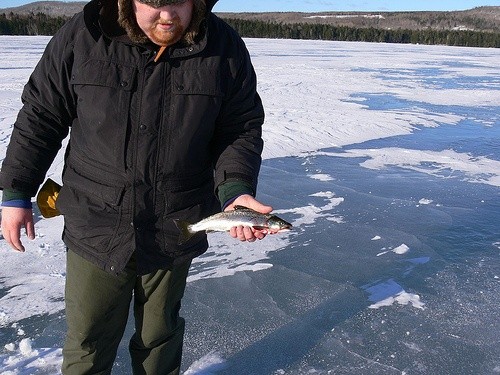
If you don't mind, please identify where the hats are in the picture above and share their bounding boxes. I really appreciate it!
[117,0,206,45]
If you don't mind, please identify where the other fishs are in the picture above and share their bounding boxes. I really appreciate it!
[172,205,292,246]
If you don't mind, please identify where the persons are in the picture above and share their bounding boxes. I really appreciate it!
[1,0,273,375]
